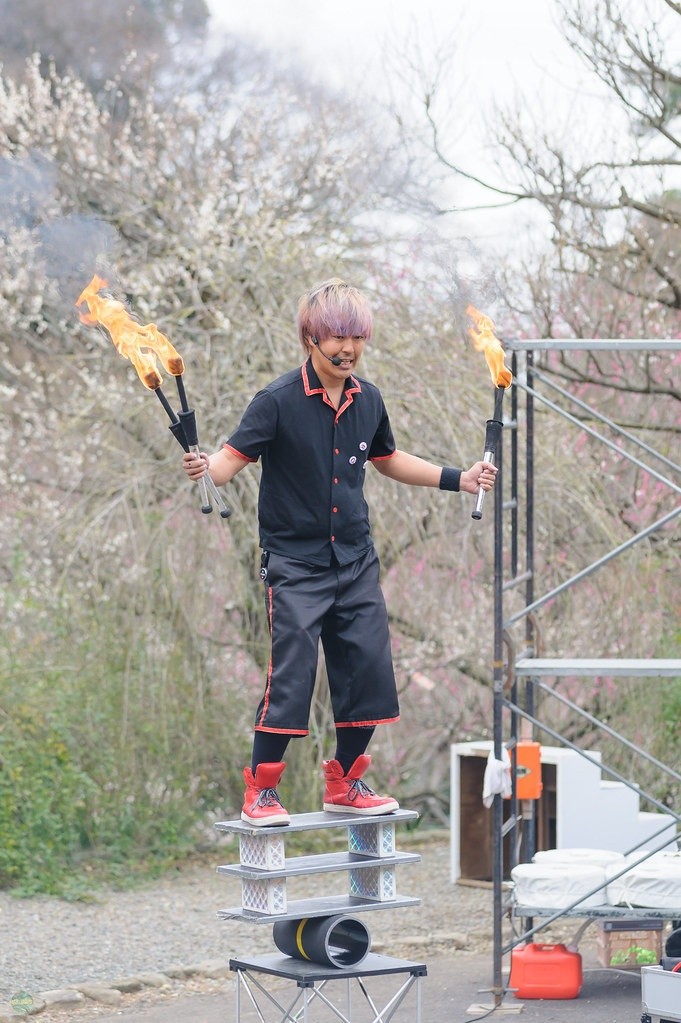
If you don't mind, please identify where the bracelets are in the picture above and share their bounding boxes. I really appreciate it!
[438,466,463,492]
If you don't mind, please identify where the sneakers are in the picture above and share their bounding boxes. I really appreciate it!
[241,762,290,826]
[323,754,399,814]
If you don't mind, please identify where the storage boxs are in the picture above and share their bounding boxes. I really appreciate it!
[509,943,583,999]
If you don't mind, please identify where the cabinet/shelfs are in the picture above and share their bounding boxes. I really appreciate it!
[213,808,429,1023]
[640,965,681,1023]
[452,739,603,891]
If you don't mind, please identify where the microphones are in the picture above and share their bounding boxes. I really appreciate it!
[311,334,341,366]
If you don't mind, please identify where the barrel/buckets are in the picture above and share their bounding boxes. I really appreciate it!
[510,942,583,1000]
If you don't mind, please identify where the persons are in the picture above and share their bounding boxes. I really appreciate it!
[181,277,500,828]
